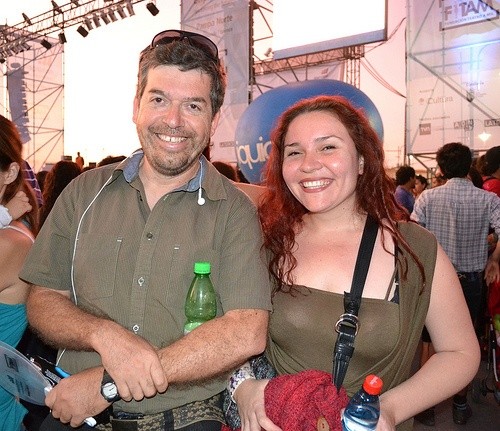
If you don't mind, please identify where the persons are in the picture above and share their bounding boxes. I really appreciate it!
[393,144,500,359]
[222,97,481,431]
[21,152,127,234]
[414,142,500,426]
[0,190,33,232]
[18,38,275,431]
[0,113,36,431]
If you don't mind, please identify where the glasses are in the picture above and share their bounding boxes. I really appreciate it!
[151,29,221,68]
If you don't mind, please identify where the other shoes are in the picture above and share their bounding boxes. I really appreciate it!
[414,411,435,426]
[452,404,472,425]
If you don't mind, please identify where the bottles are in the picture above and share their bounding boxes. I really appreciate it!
[342,375,384,431]
[183,261,217,337]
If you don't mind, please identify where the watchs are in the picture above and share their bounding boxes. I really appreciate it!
[99,369,123,404]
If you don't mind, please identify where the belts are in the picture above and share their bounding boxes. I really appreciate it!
[97,391,227,431]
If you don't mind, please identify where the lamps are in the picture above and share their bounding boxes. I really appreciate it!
[92,12,102,28]
[125,0,136,17]
[100,9,111,25]
[116,3,127,20]
[40,34,52,49]
[77,24,88,38]
[146,0,159,16]
[84,19,93,31]
[0,38,30,63]
[106,7,118,22]
[58,27,67,44]
